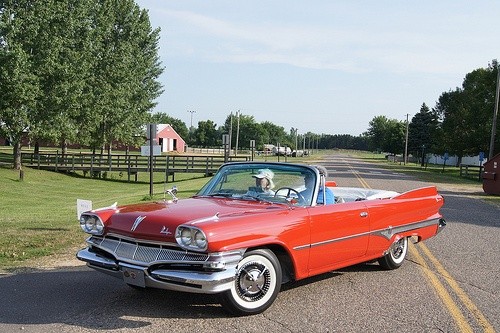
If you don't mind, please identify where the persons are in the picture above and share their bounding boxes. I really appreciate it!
[251,169,275,197]
[298,174,334,204]
[258,152,260,155]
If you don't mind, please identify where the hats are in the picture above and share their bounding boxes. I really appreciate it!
[252,169,274,179]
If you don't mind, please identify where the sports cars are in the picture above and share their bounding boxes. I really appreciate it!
[75,161,446,316]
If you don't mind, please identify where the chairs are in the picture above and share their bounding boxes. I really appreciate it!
[334,194,344,204]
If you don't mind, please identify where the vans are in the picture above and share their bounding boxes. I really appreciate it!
[265,145,310,157]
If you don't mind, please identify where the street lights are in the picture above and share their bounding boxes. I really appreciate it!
[187,110,198,127]
